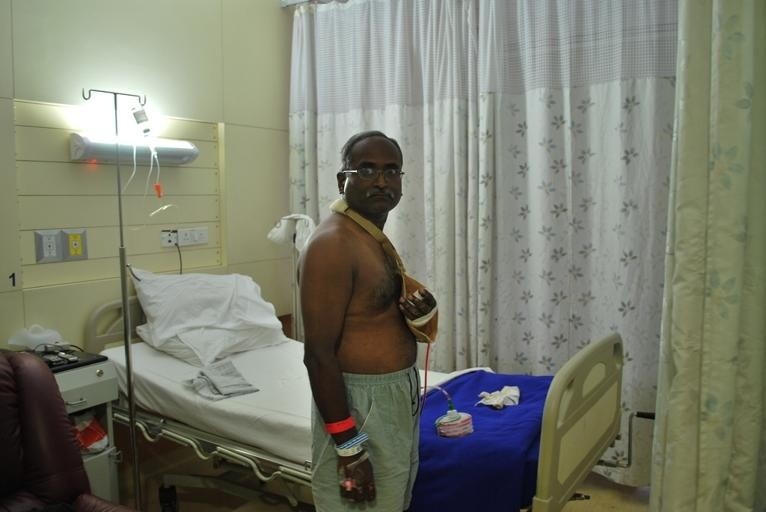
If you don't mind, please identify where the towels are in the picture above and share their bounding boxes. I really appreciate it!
[183,361,261,399]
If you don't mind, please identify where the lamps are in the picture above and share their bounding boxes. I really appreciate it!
[69,132,199,165]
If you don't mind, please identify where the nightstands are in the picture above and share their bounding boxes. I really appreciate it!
[41,345,121,505]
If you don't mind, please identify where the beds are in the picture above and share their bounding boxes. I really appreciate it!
[83,295,623,512]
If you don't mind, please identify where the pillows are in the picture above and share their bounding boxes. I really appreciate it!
[135,322,289,367]
[129,265,283,348]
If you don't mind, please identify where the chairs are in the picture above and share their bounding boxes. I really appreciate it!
[0,348,134,512]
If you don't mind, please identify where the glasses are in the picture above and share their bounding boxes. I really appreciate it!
[342,168,405,181]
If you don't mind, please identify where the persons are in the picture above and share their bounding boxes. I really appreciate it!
[298,130,437,512]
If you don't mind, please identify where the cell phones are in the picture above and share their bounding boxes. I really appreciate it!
[43,353,62,365]
[58,350,78,362]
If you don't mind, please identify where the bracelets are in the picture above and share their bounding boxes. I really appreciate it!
[324,416,370,469]
